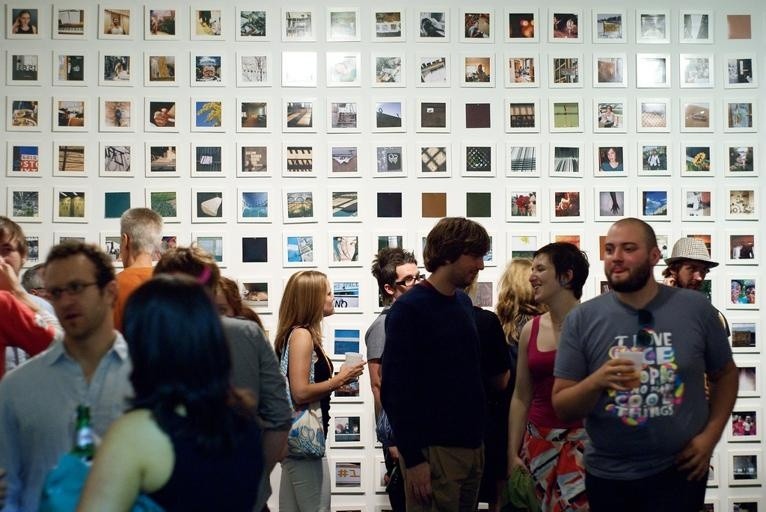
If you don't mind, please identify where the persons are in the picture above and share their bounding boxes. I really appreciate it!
[599,105,618,128]
[153,104,175,126]
[559,193,571,210]
[274,270,367,512]
[742,415,754,435]
[365,247,422,512]
[647,149,661,170]
[496,256,551,359]
[333,236,357,263]
[0,290,57,379]
[507,240,590,512]
[744,284,755,304]
[335,423,345,434]
[114,207,165,330]
[661,237,731,401]
[379,216,492,512]
[0,241,137,512]
[152,246,294,511]
[0,215,65,372]
[609,192,620,215]
[214,276,264,332]
[349,419,360,434]
[74,278,272,512]
[731,280,743,304]
[601,147,623,172]
[13,9,37,34]
[106,17,126,34]
[550,217,740,512]
[469,63,490,82]
[473,304,514,389]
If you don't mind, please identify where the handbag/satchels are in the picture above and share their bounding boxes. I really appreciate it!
[278,327,325,459]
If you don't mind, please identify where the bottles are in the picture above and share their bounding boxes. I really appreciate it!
[68,405,96,462]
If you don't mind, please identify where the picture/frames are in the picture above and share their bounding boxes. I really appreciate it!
[677,9,713,45]
[416,143,451,177]
[735,362,761,398]
[190,186,227,224]
[548,187,584,222]
[52,187,89,224]
[370,143,407,177]
[591,97,628,132]
[332,504,367,512]
[504,6,539,42]
[415,98,452,134]
[473,279,498,313]
[415,8,451,43]
[326,97,362,135]
[281,141,316,178]
[728,451,762,488]
[724,53,759,89]
[281,51,311,87]
[374,276,391,314]
[190,4,225,41]
[644,230,673,267]
[682,188,715,222]
[635,9,670,44]
[594,187,629,221]
[327,187,363,223]
[190,143,226,179]
[370,6,406,42]
[326,50,356,87]
[370,53,408,89]
[236,141,271,178]
[506,232,539,267]
[234,51,272,87]
[96,49,134,86]
[98,139,135,178]
[97,96,137,133]
[99,231,127,269]
[636,52,671,89]
[594,274,613,299]
[143,142,181,178]
[52,139,89,177]
[373,454,391,495]
[374,503,394,512]
[324,279,365,312]
[6,140,43,177]
[477,237,498,267]
[458,53,495,88]
[547,6,585,45]
[549,52,581,87]
[723,188,759,220]
[6,187,44,223]
[699,498,720,512]
[52,49,89,88]
[281,7,316,41]
[678,96,714,133]
[237,186,272,223]
[459,6,497,43]
[679,52,714,89]
[636,142,672,175]
[699,448,719,489]
[549,141,583,178]
[725,145,757,177]
[591,53,623,87]
[142,51,180,87]
[189,51,225,88]
[680,142,714,176]
[681,231,717,267]
[503,52,539,89]
[329,370,363,404]
[329,412,366,448]
[191,232,226,268]
[325,326,362,361]
[5,95,44,132]
[461,143,497,178]
[726,404,762,443]
[727,496,766,512]
[144,96,180,133]
[235,6,272,42]
[593,143,627,177]
[590,6,628,44]
[504,98,541,133]
[725,233,757,266]
[143,4,181,41]
[414,54,450,89]
[504,143,541,177]
[638,187,670,223]
[369,98,407,133]
[722,98,759,132]
[281,187,318,224]
[506,187,541,222]
[241,277,274,314]
[549,94,585,133]
[636,97,670,133]
[19,232,43,270]
[5,3,42,40]
[416,233,429,269]
[144,187,183,223]
[329,458,365,493]
[326,7,359,44]
[281,97,316,133]
[328,231,363,267]
[549,232,585,253]
[5,50,44,87]
[236,96,271,133]
[726,318,761,354]
[50,3,86,40]
[53,232,88,257]
[282,231,318,266]
[147,231,181,266]
[190,95,226,133]
[725,275,760,311]
[328,143,353,180]
[52,95,88,133]
[97,3,135,40]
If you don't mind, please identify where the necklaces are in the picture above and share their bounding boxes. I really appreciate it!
[550,300,580,327]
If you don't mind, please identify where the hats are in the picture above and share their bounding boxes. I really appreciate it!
[664,237,719,268]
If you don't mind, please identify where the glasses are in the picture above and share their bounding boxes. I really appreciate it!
[48,278,98,299]
[636,307,653,347]
[396,274,425,287]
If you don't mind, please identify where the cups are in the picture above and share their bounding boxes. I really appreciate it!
[615,352,642,388]
[344,352,363,382]
